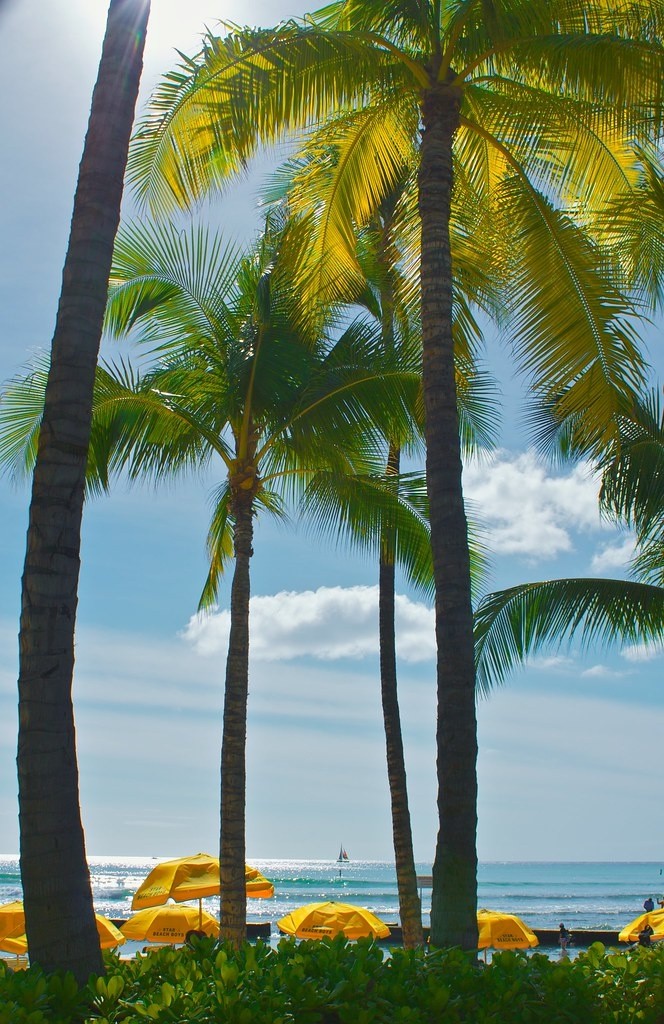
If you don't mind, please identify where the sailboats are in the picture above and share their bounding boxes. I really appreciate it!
[336,843,350,862]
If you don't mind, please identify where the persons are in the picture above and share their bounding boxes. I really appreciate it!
[660,869,662,875]
[644,896,664,913]
[559,923,573,957]
[183,929,207,945]
[478,958,493,965]
[638,924,654,947]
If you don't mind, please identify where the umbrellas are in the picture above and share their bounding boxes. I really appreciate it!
[0,899,126,966]
[118,905,220,948]
[131,853,274,932]
[276,901,391,942]
[618,908,664,942]
[427,909,539,966]
